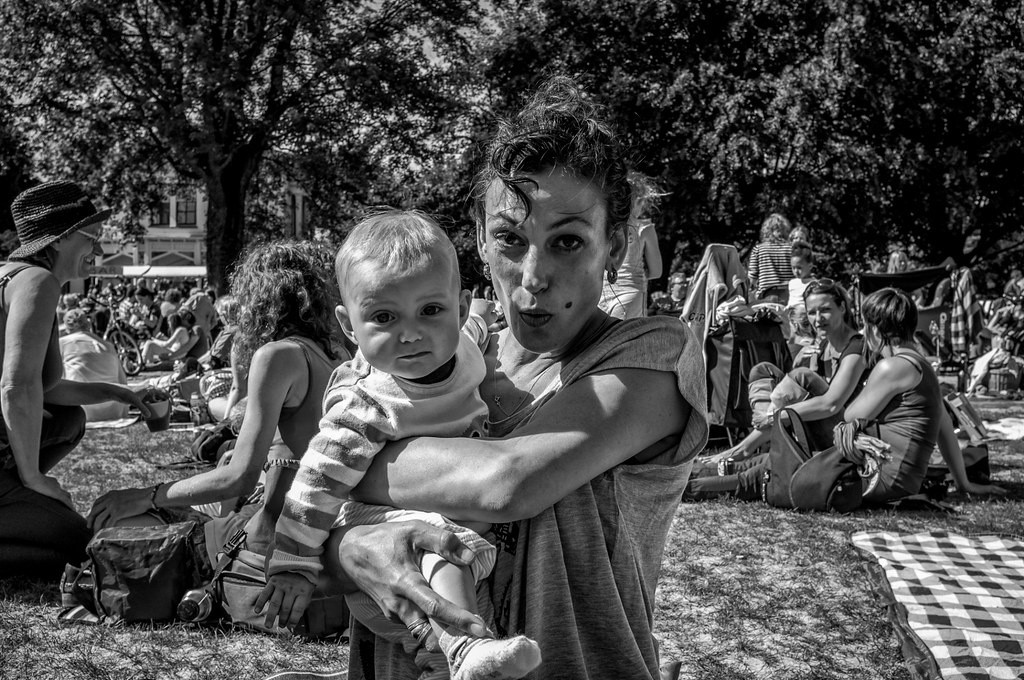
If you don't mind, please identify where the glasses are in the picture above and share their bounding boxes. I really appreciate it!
[803,279,843,298]
[672,281,689,288]
[76,229,104,245]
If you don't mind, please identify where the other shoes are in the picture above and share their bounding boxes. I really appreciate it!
[59,560,95,609]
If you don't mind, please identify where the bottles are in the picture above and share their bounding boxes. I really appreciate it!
[958,353,967,392]
[177,585,212,621]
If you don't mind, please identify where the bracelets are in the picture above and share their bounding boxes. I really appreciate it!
[151,482,169,512]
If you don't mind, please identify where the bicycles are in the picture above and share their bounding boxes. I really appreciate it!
[89,286,144,375]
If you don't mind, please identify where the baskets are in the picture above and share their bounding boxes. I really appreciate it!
[983,364,1023,393]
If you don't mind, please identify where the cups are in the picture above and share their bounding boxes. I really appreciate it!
[143,400,170,432]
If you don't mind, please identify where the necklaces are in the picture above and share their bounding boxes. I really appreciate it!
[494,331,590,417]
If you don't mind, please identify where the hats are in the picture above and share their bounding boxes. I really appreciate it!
[9,180,113,259]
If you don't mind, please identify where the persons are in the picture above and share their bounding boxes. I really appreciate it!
[596,166,663,320]
[473,283,497,304]
[0,179,178,571]
[87,237,354,639]
[57,275,257,428]
[319,75,708,680]
[254,208,542,680]
[647,213,1024,510]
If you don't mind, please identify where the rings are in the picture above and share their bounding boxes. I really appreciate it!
[406,618,432,644]
[144,388,150,394]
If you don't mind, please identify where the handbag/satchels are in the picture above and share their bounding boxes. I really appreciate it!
[769,406,863,513]
[86,506,214,629]
[1002,277,1024,304]
[917,445,991,509]
